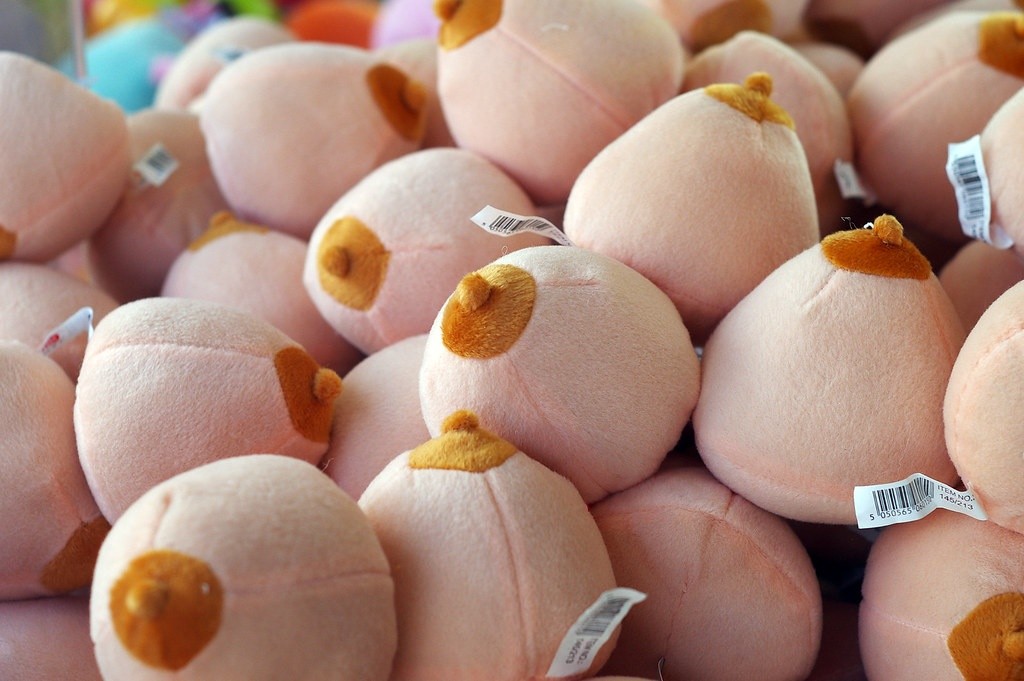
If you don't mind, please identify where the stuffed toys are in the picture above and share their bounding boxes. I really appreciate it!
[1,2,1023,681]
